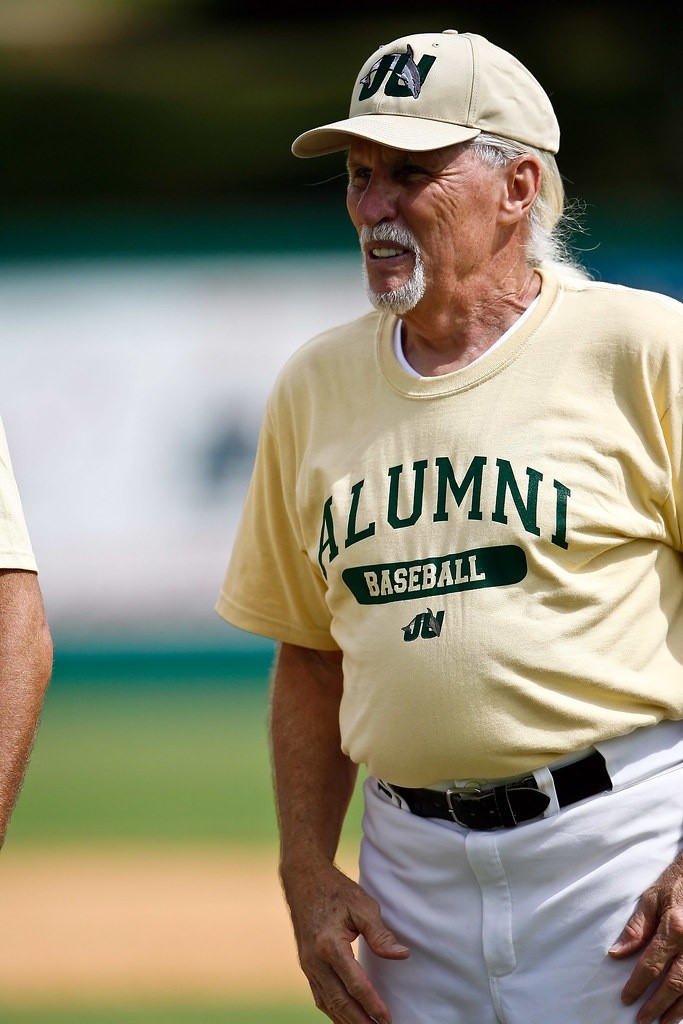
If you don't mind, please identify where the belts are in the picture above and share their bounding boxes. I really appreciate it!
[378,751,612,832]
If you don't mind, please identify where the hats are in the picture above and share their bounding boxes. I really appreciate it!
[290,29,561,160]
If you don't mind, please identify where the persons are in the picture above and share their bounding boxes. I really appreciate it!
[213,29,683,1024]
[0,392,56,854]
